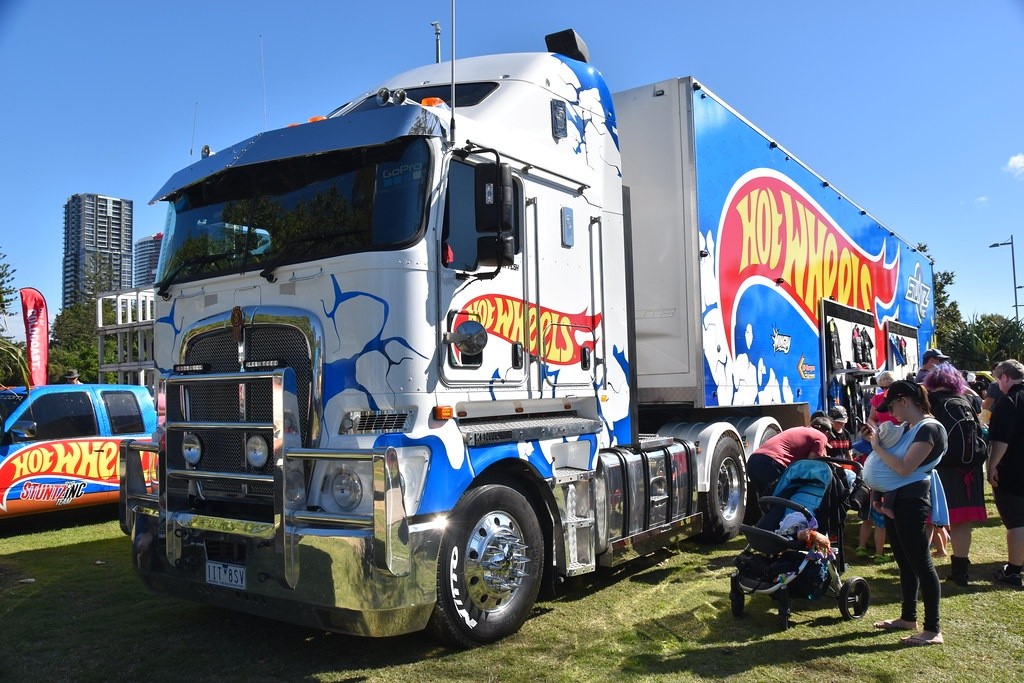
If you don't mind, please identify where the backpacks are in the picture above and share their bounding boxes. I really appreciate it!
[930,393,988,466]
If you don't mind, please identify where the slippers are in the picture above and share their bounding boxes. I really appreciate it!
[898,635,944,645]
[874,620,920,631]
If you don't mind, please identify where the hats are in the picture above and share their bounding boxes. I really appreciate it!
[967,373,976,383]
[812,416,837,439]
[922,348,950,359]
[875,380,918,412]
[829,405,848,424]
[851,437,873,454]
[878,421,904,449]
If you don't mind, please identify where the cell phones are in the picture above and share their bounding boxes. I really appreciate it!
[855,417,871,435]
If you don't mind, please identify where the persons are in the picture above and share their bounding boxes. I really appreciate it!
[860,380,947,645]
[987,359,1024,587]
[65,369,82,384]
[871,420,904,518]
[747,349,1003,586]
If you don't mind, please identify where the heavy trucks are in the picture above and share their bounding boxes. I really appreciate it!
[119,27,940,648]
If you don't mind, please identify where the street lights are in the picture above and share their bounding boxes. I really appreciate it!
[988,234,1024,324]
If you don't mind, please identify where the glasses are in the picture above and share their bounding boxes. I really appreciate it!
[880,386,889,390]
[887,398,902,411]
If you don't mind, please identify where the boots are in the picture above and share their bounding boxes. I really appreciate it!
[945,555,970,590]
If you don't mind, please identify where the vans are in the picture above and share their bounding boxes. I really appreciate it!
[0,384,158,519]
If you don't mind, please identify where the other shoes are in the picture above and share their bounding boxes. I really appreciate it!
[992,564,1024,591]
[874,555,891,564]
[856,547,869,557]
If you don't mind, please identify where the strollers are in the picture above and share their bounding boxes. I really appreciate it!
[729,456,871,624]
